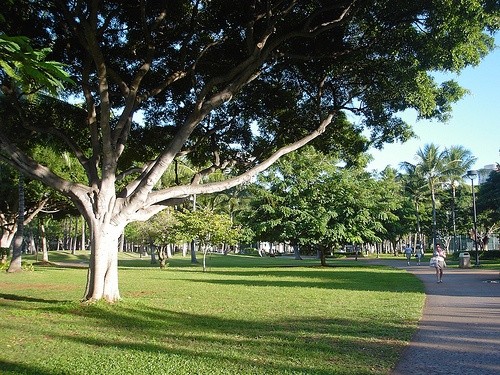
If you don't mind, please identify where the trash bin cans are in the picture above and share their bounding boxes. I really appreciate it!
[458,251,471,267]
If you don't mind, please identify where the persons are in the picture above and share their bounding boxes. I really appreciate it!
[158,244,167,269]
[415,244,422,266]
[432,243,446,283]
[403,243,413,266]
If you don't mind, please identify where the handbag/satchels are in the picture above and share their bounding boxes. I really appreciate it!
[430,257,438,267]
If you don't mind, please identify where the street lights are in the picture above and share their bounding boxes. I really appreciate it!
[466,170,480,266]
